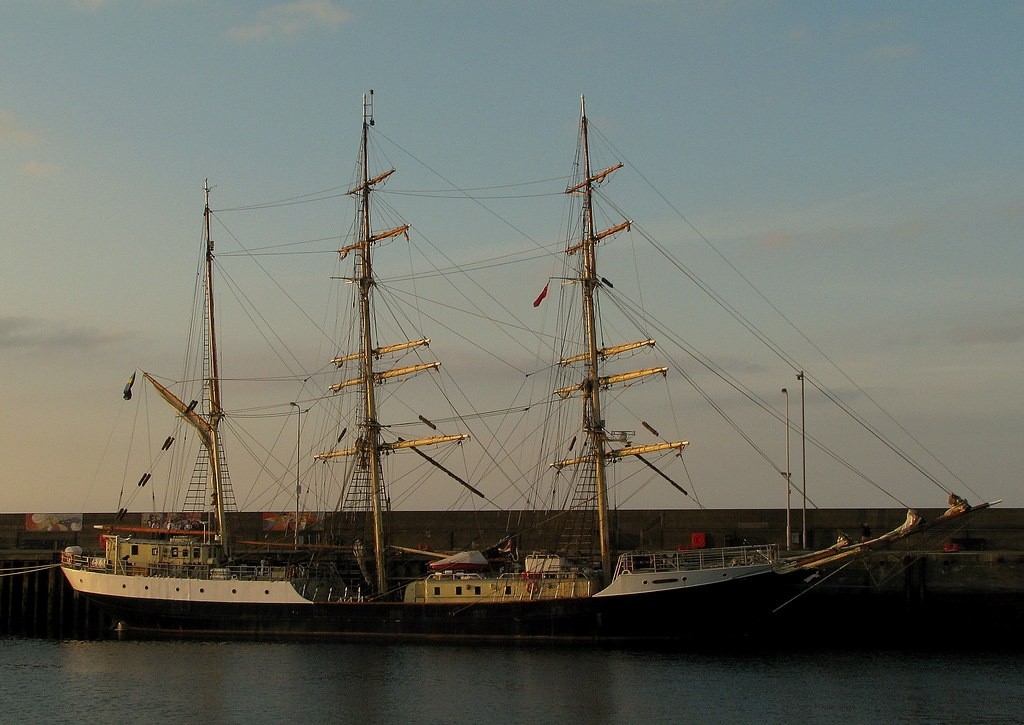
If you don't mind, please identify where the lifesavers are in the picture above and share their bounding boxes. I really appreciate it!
[526,582,539,594]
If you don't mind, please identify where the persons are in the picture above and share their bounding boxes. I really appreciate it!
[150,519,193,540]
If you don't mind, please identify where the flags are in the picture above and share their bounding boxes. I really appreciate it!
[533,285,547,307]
[123,372,135,400]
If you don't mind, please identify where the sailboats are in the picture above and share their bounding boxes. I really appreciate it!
[55,83,1002,640]
